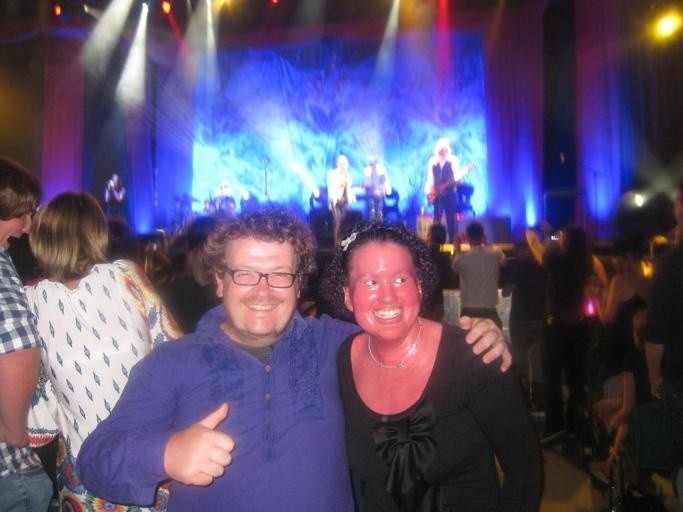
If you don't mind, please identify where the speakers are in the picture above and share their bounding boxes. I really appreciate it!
[543,191,578,234]
[492,216,510,242]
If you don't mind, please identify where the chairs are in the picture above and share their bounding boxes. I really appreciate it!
[605,395,676,510]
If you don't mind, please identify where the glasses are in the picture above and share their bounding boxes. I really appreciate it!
[222,263,303,289]
